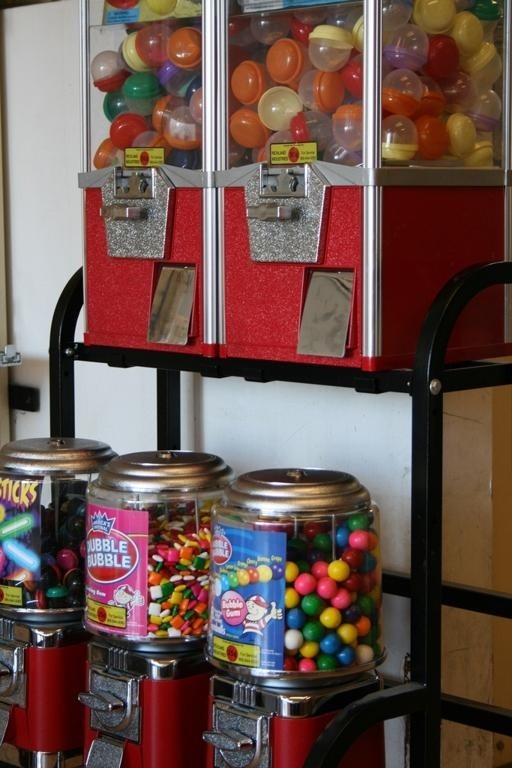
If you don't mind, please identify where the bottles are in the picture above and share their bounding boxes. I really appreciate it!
[210,467,387,687]
[0,436,117,622]
[84,449,234,653]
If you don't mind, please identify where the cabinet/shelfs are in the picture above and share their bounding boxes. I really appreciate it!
[48,262,511,768]
